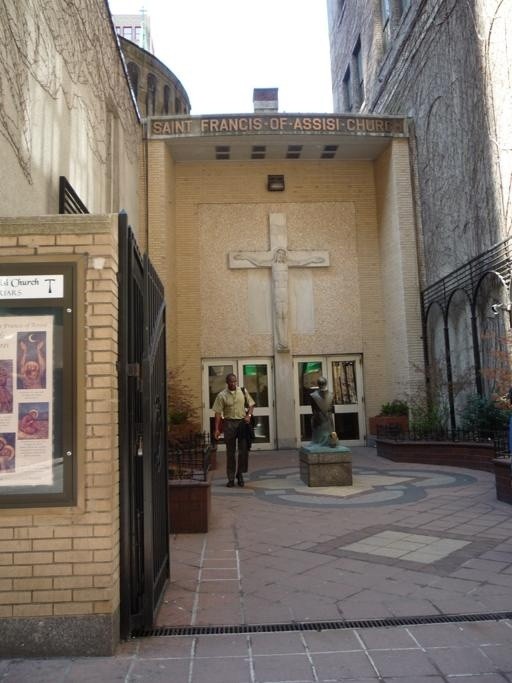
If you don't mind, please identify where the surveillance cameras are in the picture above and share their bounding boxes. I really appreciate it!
[492,303,511,315]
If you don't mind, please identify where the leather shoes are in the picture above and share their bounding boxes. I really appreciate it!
[226,480,234,487]
[236,473,244,487]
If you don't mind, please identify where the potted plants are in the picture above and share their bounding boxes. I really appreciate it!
[373,401,409,434]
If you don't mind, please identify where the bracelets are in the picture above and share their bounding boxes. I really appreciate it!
[247,413,252,417]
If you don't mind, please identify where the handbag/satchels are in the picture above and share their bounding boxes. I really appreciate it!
[241,387,259,429]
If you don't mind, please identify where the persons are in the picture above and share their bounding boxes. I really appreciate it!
[309,376,335,445]
[0,335,47,470]
[211,373,256,487]
[234,248,325,349]
